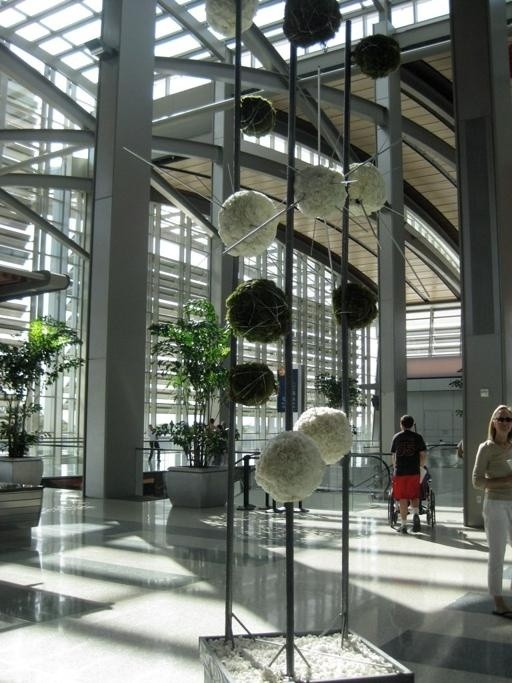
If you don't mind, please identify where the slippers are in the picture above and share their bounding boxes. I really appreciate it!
[492,610,512,620]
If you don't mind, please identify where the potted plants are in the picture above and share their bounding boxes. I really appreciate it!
[0,315,86,484]
[148,298,239,506]
[315,372,368,490]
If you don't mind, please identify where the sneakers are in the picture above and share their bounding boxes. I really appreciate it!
[413,513,420,532]
[397,525,408,534]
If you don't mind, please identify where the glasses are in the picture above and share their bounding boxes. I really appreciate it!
[496,417,512,422]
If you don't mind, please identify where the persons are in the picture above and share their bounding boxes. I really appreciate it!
[472,404,512,619]
[455,440,465,467]
[210,418,228,466]
[391,415,427,534]
[147,425,160,461]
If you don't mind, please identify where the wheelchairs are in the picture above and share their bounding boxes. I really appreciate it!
[384,466,441,532]
